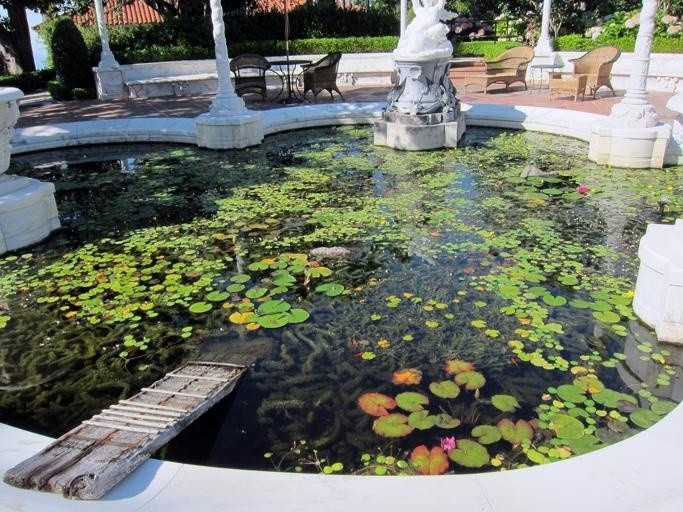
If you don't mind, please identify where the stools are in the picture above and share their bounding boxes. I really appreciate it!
[548,72,587,102]
[462,75,508,94]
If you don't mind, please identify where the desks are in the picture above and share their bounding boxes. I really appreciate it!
[531,65,564,90]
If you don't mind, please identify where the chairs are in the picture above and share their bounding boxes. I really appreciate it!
[483,46,535,94]
[567,44,621,99]
[229,52,345,105]
